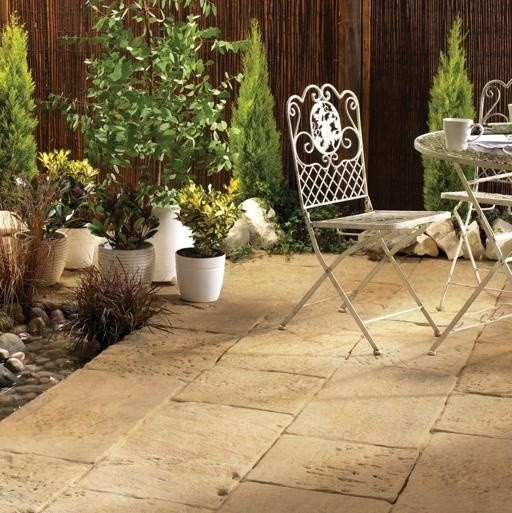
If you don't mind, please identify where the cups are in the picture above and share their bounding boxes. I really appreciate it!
[442,117,484,153]
